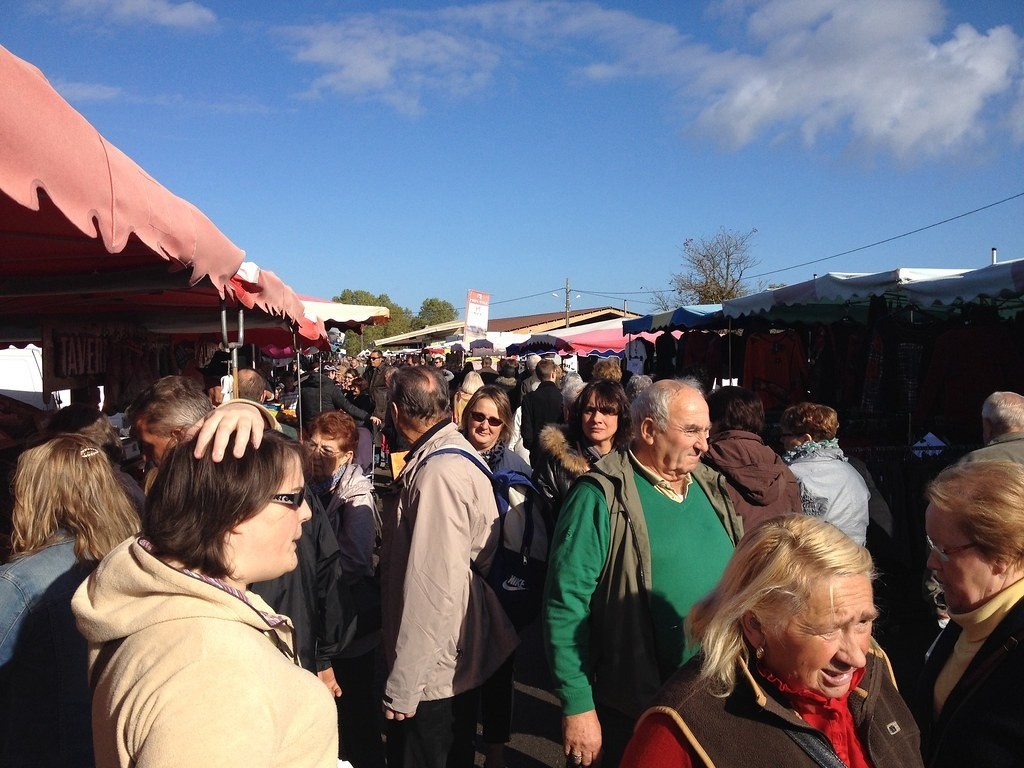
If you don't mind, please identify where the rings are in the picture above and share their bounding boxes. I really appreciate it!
[574,755,582,760]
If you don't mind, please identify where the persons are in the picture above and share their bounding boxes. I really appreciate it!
[0,349,1024,768]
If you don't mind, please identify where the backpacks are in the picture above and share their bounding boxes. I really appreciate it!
[412,448,556,626]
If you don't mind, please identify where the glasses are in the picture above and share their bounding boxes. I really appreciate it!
[269,487,306,509]
[925,536,976,562]
[370,357,379,361]
[434,362,438,364]
[469,410,503,427]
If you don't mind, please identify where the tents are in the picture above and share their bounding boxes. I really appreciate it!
[433,257,1024,435]
[0,50,390,439]
